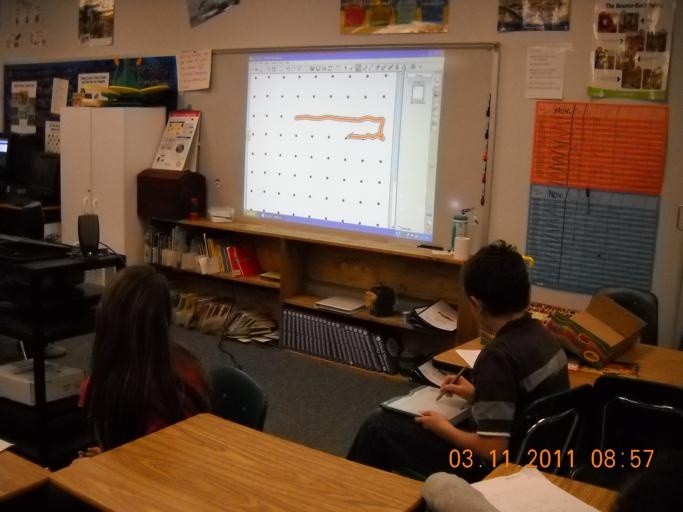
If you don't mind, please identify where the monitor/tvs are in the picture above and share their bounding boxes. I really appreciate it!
[30,150,60,201]
[0,132,20,194]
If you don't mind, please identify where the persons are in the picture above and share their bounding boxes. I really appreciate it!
[78,266,211,459]
[345,244,570,473]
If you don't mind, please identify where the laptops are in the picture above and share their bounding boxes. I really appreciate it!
[0,233,74,263]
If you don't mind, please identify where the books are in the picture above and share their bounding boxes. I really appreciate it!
[144,226,262,279]
[170,286,404,375]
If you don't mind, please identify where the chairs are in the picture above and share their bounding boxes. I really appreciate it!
[512,375,680,478]
[594,287,659,345]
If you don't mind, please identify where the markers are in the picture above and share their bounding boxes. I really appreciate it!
[480,92,492,208]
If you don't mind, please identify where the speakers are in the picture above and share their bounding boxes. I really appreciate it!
[137,169,206,221]
[77,212,101,261]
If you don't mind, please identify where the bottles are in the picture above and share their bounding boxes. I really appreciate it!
[450,214,468,254]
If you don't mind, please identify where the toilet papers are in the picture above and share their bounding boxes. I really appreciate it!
[454,235,473,262]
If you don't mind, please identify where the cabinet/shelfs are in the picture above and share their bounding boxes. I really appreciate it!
[0,237,127,464]
[139,212,512,377]
[60,105,169,264]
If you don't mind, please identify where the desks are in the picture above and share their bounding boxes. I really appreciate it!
[49,410,426,510]
[433,313,679,393]
[1,443,52,510]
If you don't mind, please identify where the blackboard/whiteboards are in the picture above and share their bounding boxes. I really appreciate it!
[178,41,500,258]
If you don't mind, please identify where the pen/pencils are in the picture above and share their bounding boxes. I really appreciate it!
[435,366,467,402]
[418,244,445,250]
